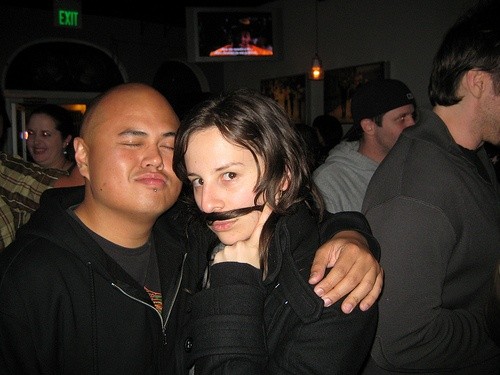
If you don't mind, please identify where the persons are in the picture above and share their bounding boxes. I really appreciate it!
[0,82,385,375]
[310,78,416,214]
[171,87,379,375]
[360,5,500,375]
[26,104,84,183]
[0,152,84,247]
[209,18,273,56]
[292,115,344,174]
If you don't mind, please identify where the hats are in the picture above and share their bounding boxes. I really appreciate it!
[341,79,416,140]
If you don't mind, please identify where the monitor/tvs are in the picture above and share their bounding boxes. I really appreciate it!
[185,7,285,62]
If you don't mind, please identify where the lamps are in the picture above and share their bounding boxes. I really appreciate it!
[310,1,325,80]
